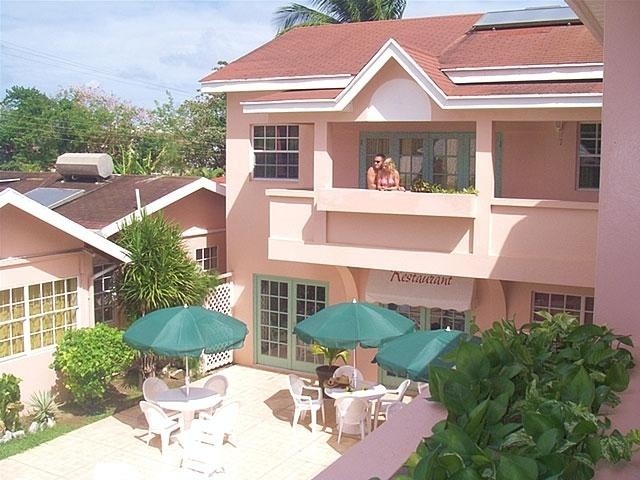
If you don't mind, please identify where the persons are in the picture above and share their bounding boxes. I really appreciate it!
[366,152,400,189]
[376,157,405,192]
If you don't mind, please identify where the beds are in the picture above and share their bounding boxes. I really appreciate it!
[369,325,483,385]
[290,297,417,387]
[122,302,249,395]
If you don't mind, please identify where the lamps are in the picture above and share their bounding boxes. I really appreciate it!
[287,364,410,446]
[138,375,243,477]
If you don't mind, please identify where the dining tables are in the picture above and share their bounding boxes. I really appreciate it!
[306,341,350,400]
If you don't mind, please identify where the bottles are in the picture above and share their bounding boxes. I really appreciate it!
[336,375,350,384]
[322,377,339,388]
